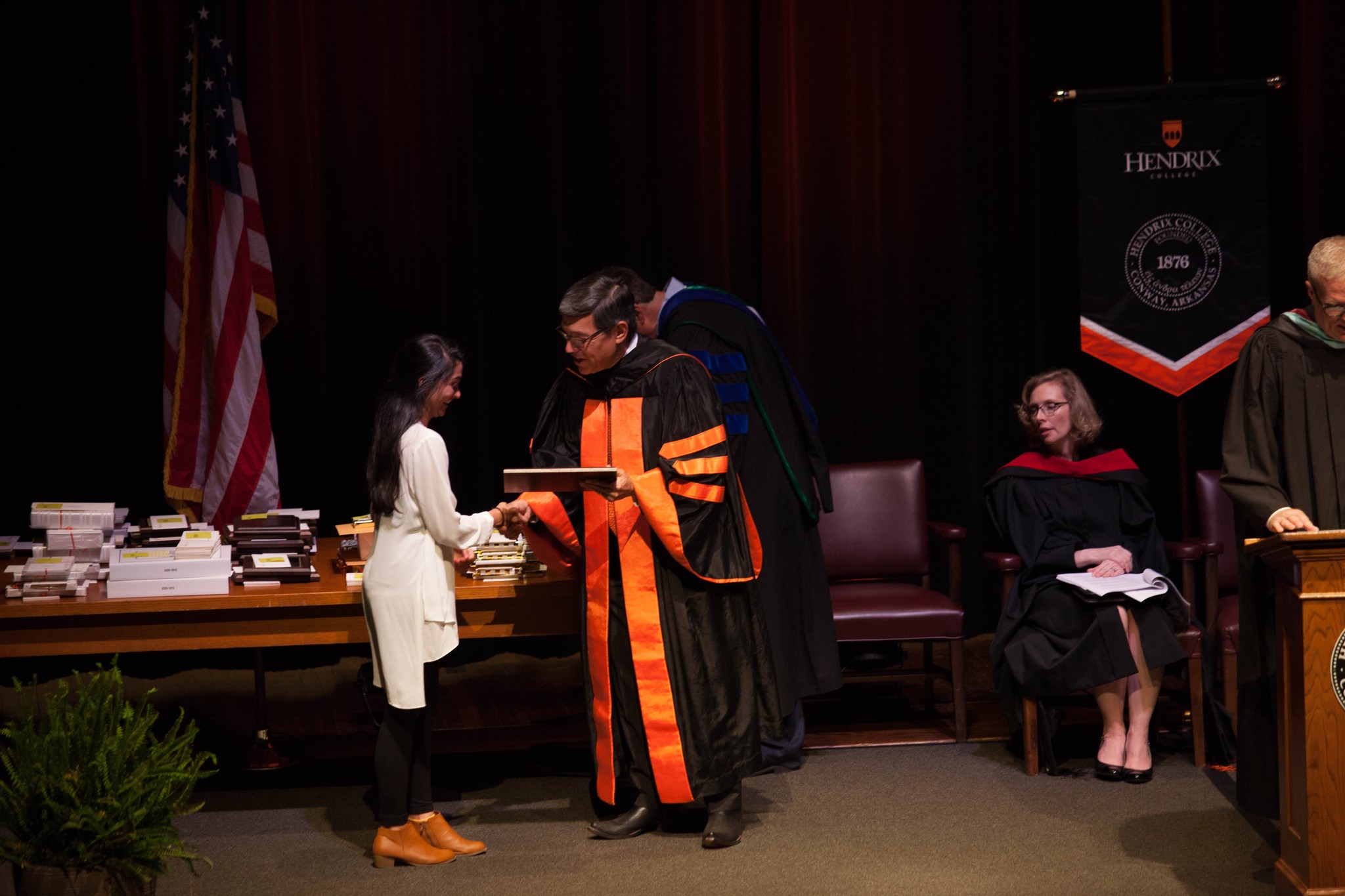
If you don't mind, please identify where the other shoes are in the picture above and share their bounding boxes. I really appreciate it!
[741,752,802,776]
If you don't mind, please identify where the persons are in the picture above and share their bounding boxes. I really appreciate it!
[501,278,761,848]
[629,268,833,776]
[364,334,521,868]
[979,368,1174,783]
[1223,236,1345,870]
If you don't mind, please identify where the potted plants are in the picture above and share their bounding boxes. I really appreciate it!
[0,653,219,896]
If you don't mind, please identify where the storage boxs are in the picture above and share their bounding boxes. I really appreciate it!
[0,502,238,599]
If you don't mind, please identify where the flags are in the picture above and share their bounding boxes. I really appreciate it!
[1069,78,1269,477]
[163,0,279,525]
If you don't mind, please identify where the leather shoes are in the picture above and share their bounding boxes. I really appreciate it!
[587,804,698,838]
[1095,745,1122,780]
[1123,750,1154,783]
[702,790,745,847]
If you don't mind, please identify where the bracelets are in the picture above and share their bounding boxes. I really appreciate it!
[494,507,504,528]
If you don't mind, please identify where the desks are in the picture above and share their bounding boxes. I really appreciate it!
[0,524,602,775]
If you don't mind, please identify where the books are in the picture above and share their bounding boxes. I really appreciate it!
[0,503,547,602]
[1055,568,1189,603]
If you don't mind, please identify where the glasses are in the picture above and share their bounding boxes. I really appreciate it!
[1311,283,1345,317]
[555,323,615,348]
[1029,401,1069,417]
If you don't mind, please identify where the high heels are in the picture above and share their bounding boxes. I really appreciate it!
[406,810,487,856]
[373,824,457,868]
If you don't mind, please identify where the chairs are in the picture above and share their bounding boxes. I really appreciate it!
[1195,471,1241,763]
[813,459,967,742]
[983,539,1209,777]
